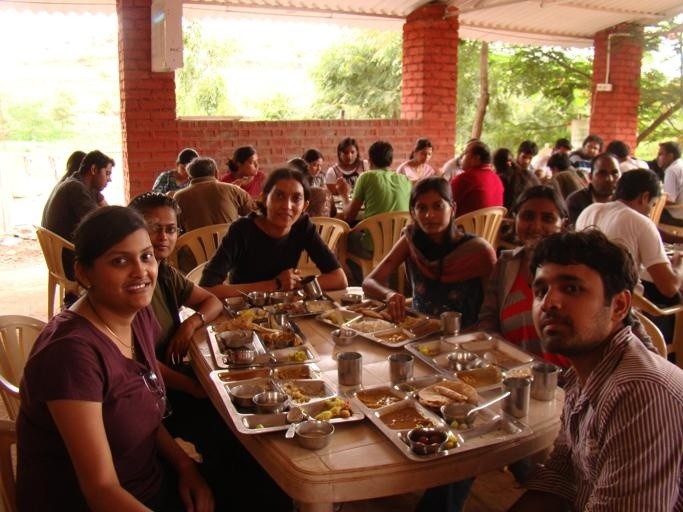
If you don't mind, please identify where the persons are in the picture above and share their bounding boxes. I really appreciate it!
[128,194,223,477]
[438,154,466,182]
[202,169,349,300]
[301,148,327,189]
[516,141,548,185]
[537,139,571,180]
[506,231,682,512]
[153,148,199,198]
[333,142,414,285]
[557,154,622,236]
[657,142,683,226]
[547,153,583,202]
[59,152,107,207]
[220,147,266,201]
[451,139,505,220]
[42,151,114,307]
[17,206,216,512]
[288,158,337,217]
[399,139,437,185]
[492,150,540,213]
[363,177,496,322]
[415,183,569,512]
[179,158,253,264]
[574,168,683,297]
[606,141,650,175]
[570,137,602,181]
[323,138,369,195]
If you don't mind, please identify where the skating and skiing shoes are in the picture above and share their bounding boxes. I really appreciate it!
[142,371,173,419]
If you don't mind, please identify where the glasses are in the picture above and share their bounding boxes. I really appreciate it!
[147,225,180,236]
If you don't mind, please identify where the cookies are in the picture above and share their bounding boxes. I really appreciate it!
[360,308,393,321]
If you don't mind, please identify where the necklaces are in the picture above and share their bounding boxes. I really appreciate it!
[86,294,135,351]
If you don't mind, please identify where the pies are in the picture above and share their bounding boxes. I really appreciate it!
[421,379,484,409]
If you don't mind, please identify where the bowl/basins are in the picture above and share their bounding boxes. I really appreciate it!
[253,392,289,412]
[224,297,244,308]
[340,294,361,306]
[331,328,358,345]
[407,427,449,455]
[222,351,256,366]
[296,420,335,450]
[288,288,304,302]
[440,403,477,428]
[248,291,265,306]
[230,384,265,407]
[220,330,254,349]
[448,352,475,371]
[270,292,286,303]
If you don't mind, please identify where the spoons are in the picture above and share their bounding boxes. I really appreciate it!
[483,352,509,371]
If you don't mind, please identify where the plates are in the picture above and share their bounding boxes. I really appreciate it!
[209,363,365,434]
[205,316,320,369]
[222,289,340,319]
[351,372,533,463]
[404,329,563,394]
[315,297,440,348]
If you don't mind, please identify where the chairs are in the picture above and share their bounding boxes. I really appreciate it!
[347,211,413,297]
[0,314,49,510]
[296,215,351,278]
[453,206,507,246]
[630,291,683,358]
[165,221,232,275]
[33,223,80,320]
[653,192,668,227]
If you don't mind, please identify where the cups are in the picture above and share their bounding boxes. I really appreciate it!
[337,351,363,386]
[500,373,531,418]
[388,353,414,384]
[530,362,563,401]
[300,275,322,299]
[441,311,462,335]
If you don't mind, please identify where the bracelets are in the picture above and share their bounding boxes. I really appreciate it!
[196,312,206,326]
[275,277,283,291]
[382,289,394,303]
[342,198,348,201]
